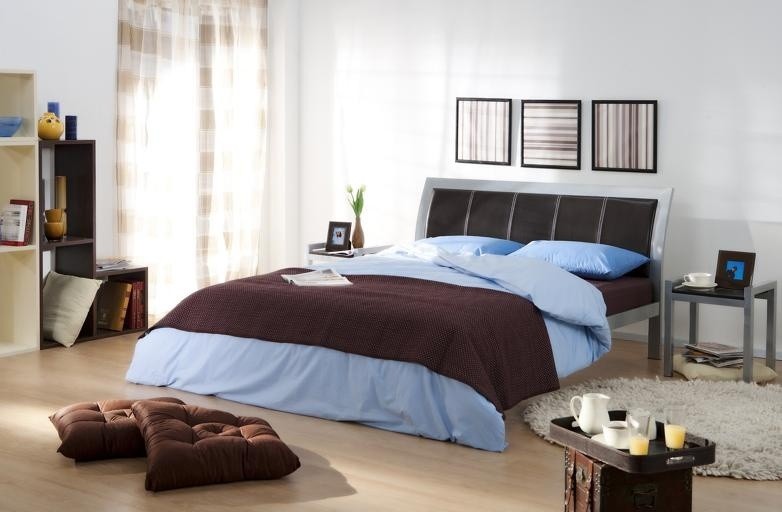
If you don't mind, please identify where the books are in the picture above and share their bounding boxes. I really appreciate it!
[684,343,743,369]
[280,267,354,287]
[96,276,145,333]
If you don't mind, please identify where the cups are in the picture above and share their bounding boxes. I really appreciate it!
[602,420,639,446]
[44,207,63,223]
[664,407,689,452]
[44,222,65,241]
[628,414,650,457]
[570,393,610,435]
[683,272,713,285]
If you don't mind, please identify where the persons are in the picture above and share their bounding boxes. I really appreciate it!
[726,266,737,280]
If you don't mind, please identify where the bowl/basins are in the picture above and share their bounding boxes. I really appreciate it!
[0,116,22,137]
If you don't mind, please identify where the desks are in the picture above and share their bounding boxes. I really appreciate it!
[306,244,392,264]
[662,276,778,383]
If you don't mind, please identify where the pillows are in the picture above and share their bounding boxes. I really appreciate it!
[414,235,524,256]
[49,396,185,461]
[130,400,301,491]
[41,270,103,349]
[510,240,651,281]
[673,352,780,381]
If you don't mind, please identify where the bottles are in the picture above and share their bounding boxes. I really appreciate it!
[47,102,60,119]
[65,115,77,140]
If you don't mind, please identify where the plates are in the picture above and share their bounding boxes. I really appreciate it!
[682,282,718,292]
[591,434,630,451]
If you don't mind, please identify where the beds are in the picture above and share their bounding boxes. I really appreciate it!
[125,177,675,453]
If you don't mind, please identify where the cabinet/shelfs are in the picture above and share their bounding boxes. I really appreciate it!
[0,69,148,359]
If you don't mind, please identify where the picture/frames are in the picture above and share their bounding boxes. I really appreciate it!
[591,99,658,175]
[521,99,581,171]
[325,222,350,252]
[455,97,513,166]
[714,249,756,287]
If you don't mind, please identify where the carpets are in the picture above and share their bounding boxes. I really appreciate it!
[523,377,782,481]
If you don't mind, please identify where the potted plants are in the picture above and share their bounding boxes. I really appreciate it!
[344,184,366,248]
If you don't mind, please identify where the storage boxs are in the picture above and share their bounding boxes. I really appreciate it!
[563,447,692,512]
